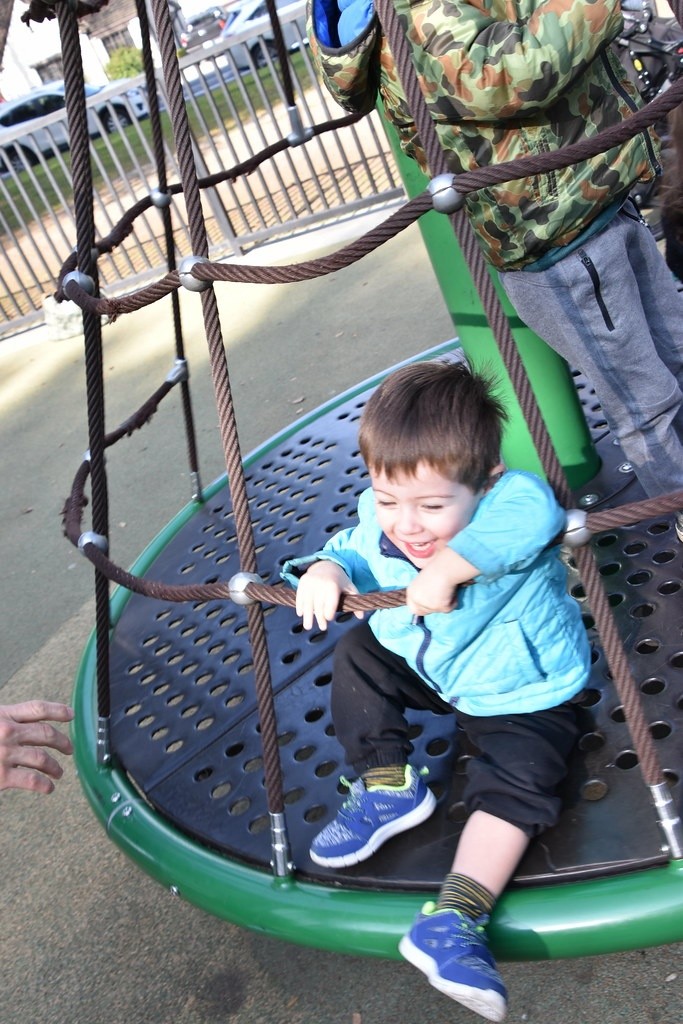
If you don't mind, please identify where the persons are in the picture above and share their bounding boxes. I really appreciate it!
[0,701,74,795]
[279,361,592,1022]
[305,1,682,539]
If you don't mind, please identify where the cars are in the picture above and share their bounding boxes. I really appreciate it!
[0,80,149,174]
[181,0,311,72]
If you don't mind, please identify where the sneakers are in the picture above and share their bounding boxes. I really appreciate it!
[308,764,436,867]
[397,901,508,1020]
